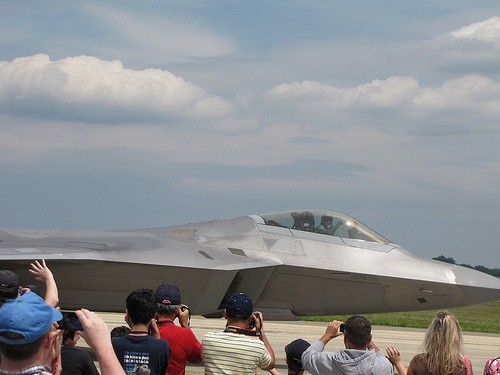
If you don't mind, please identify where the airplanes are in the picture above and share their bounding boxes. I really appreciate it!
[1,209,500,322]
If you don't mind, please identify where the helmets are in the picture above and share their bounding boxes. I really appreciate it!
[320,214,333,224]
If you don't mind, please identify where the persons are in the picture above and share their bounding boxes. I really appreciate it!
[483,357,500,375]
[111,289,171,375]
[0,292,126,375]
[60,312,100,375]
[385,311,473,375]
[300,315,394,375]
[150,283,203,375]
[197,292,276,375]
[316,214,343,236]
[111,325,130,340]
[269,339,311,375]
[0,257,59,309]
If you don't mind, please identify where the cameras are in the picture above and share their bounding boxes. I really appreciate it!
[340,324,346,333]
[250,313,259,326]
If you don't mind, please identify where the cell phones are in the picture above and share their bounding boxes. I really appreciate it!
[55,311,84,331]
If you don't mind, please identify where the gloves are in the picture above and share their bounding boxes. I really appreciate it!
[334,221,343,229]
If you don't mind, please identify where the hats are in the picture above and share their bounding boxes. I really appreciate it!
[285,338,312,363]
[0,290,63,344]
[0,269,20,292]
[226,292,253,315]
[155,284,181,305]
